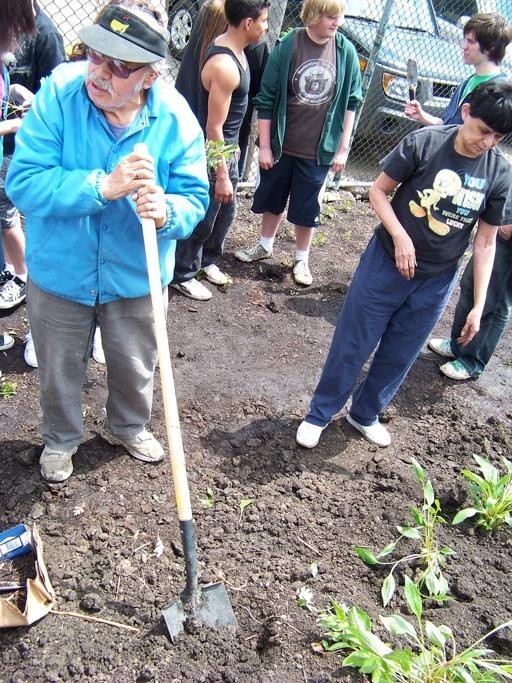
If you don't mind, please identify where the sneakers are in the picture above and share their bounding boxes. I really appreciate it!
[0,267,13,287]
[440,360,470,383]
[176,279,211,301]
[350,413,391,448]
[0,277,25,309]
[93,328,106,364]
[233,244,271,264]
[100,426,165,461]
[297,419,330,449]
[41,446,78,482]
[24,330,38,366]
[1,335,14,351]
[293,259,313,285]
[429,339,451,355]
[202,265,223,284]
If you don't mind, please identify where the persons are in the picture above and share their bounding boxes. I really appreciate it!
[169,0,269,300]
[23,0,168,368]
[0,0,37,353]
[5,5,210,483]
[428,181,512,380]
[404,11,512,127]
[0,0,66,310]
[175,0,230,119]
[235,0,364,286]
[297,79,512,448]
[238,39,269,183]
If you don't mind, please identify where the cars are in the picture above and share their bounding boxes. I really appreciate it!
[166,0,511,145]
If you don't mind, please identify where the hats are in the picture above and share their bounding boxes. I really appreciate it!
[80,8,167,62]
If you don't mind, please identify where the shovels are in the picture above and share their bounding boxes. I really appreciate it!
[134,141,240,643]
